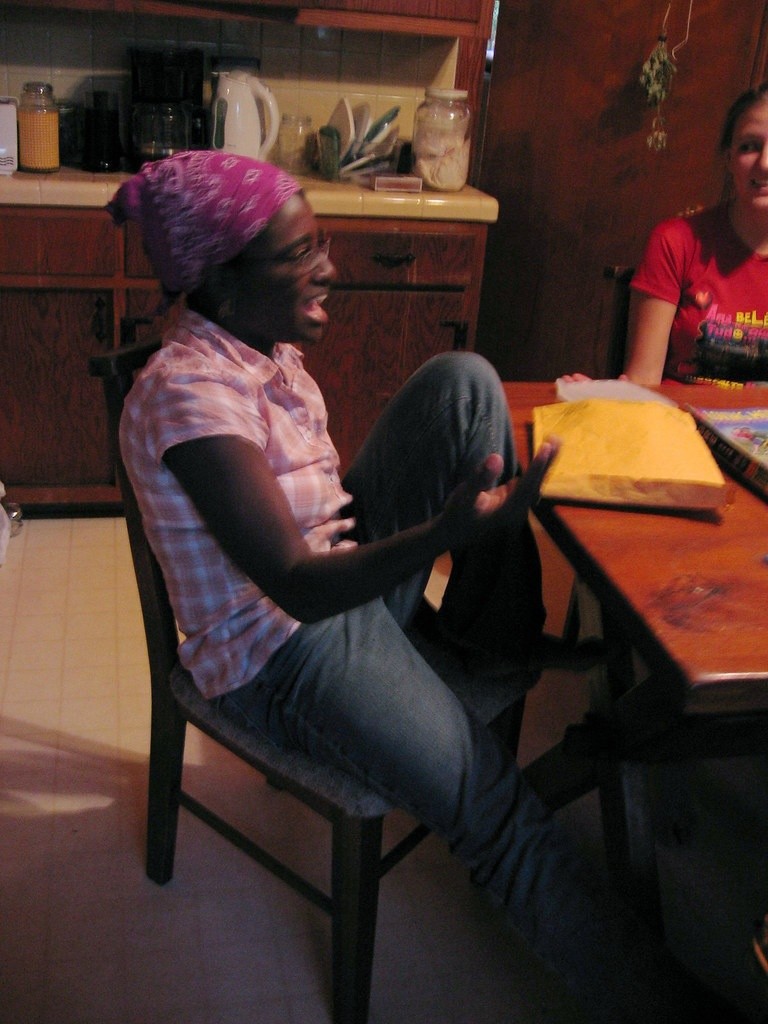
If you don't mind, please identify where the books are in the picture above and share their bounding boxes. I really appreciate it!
[686,402,768,501]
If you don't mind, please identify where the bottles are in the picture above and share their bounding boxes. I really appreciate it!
[17,82,59,171]
[411,87,471,191]
[59,101,78,160]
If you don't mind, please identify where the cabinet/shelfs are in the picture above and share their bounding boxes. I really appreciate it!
[0,203,492,509]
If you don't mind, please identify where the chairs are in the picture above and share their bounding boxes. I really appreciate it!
[89,315,547,1024]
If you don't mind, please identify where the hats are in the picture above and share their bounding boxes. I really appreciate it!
[105,149,303,302]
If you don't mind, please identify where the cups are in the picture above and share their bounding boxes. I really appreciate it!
[280,112,316,176]
[83,91,121,171]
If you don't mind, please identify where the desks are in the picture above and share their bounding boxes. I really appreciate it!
[498,380,768,898]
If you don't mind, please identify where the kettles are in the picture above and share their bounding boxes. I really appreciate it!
[210,71,279,162]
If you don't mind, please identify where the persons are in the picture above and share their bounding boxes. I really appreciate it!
[560,82,768,389]
[105,153,751,1024]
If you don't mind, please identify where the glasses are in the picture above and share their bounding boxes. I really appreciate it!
[230,238,331,272]
